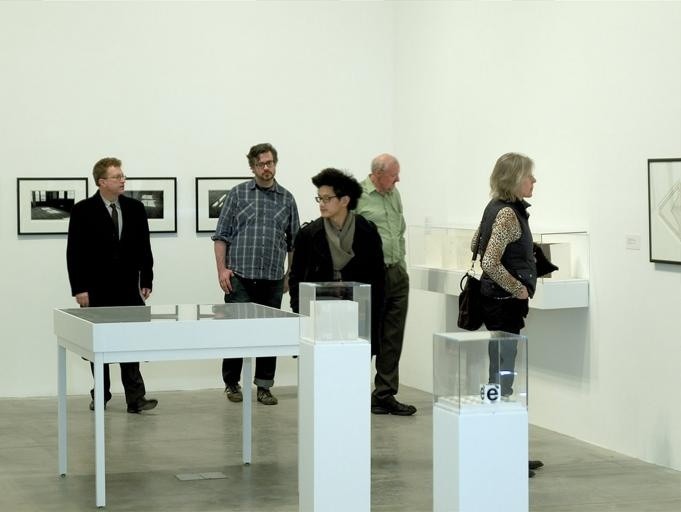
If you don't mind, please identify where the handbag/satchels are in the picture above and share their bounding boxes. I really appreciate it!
[458,273,483,331]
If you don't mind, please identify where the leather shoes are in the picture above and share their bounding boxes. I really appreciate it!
[89,400,106,410]
[128,399,157,413]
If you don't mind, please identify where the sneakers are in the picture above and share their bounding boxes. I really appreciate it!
[530,471,535,477]
[225,385,243,402]
[530,460,543,469]
[257,387,277,405]
[372,394,416,415]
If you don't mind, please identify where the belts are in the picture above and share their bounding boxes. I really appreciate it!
[386,263,396,267]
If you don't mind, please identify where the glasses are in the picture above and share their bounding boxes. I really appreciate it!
[103,174,126,180]
[315,195,336,202]
[256,161,274,167]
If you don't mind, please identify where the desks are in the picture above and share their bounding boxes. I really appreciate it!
[52,302,310,511]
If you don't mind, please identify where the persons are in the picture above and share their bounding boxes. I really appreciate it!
[354,154,420,416]
[211,142,301,406]
[456,152,547,477]
[66,157,157,414]
[288,167,386,364]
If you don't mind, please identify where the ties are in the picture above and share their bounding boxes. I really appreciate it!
[110,204,118,228]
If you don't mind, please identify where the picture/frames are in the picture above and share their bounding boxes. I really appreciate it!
[16,177,89,236]
[647,158,681,264]
[121,177,177,234]
[195,177,255,233]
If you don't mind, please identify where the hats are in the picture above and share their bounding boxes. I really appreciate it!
[534,243,558,276]
